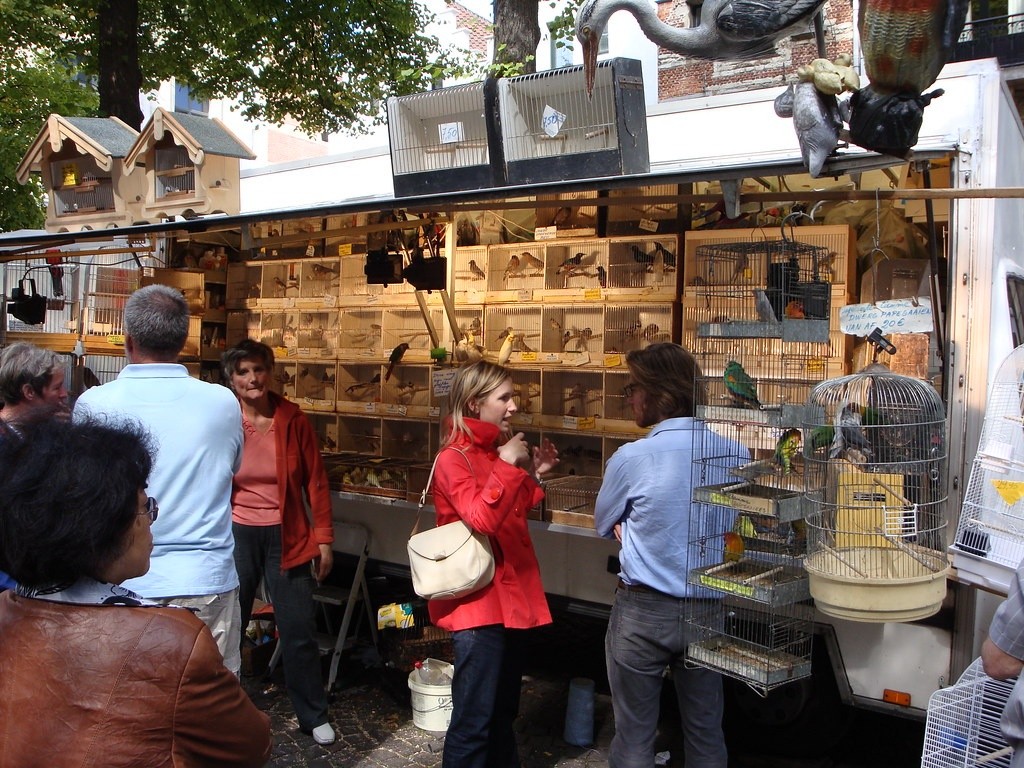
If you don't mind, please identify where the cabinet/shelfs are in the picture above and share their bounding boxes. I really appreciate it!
[135,227,855,526]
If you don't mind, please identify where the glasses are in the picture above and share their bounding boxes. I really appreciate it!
[623,381,676,399]
[132,497,159,521]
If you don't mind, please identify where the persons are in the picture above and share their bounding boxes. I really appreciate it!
[980,559,1024,766]
[428,359,560,768]
[0,408,274,768]
[594,342,753,768]
[223,339,336,744]
[0,284,244,684]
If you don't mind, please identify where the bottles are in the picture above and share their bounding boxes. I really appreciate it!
[414,661,453,686]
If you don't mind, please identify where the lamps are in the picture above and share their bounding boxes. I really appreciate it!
[404,236,448,294]
[7,277,47,325]
[365,249,403,289]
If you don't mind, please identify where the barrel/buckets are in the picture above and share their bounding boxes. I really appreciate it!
[408,665,455,732]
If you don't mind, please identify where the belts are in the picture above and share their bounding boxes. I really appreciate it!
[618,577,663,593]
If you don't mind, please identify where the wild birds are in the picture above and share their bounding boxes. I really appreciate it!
[344,384,377,400]
[769,427,802,474]
[550,318,563,335]
[468,259,486,279]
[502,254,520,282]
[522,252,544,275]
[495,327,514,343]
[805,424,835,456]
[722,361,762,410]
[830,402,888,459]
[723,531,744,563]
[498,334,515,366]
[466,337,484,362]
[456,339,467,362]
[574,0,830,102]
[241,193,675,499]
[737,511,757,538]
[384,342,409,382]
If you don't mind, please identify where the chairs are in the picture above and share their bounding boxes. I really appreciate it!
[265,523,376,691]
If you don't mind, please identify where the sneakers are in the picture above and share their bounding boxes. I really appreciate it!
[313,722,336,744]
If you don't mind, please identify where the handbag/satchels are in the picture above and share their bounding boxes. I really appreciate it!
[407,447,495,601]
